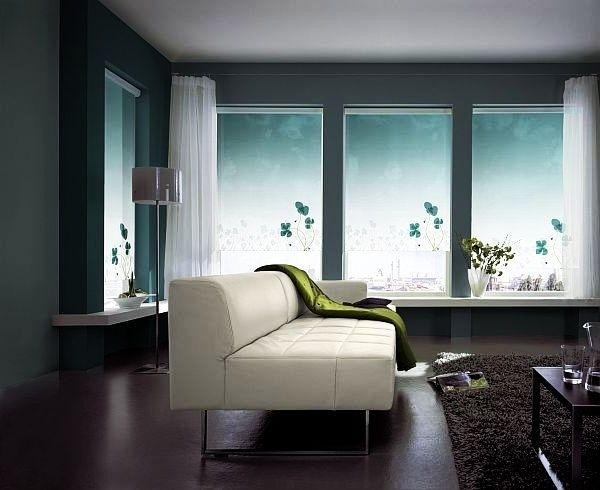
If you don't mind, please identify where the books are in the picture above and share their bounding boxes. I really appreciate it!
[426,371,490,393]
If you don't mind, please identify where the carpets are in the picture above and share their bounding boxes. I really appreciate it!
[432,351,600,490]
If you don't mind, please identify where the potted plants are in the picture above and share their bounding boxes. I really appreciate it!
[459,234,522,297]
[112,224,156,308]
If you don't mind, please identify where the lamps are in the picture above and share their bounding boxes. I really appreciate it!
[133,165,182,374]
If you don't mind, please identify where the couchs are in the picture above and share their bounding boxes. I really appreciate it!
[168,272,396,461]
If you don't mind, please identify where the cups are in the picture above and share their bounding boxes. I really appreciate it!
[467,268,491,297]
[561,345,586,384]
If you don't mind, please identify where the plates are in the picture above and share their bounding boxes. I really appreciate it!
[107,294,156,309]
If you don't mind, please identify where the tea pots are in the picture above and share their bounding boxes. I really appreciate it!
[583,322,600,393]
